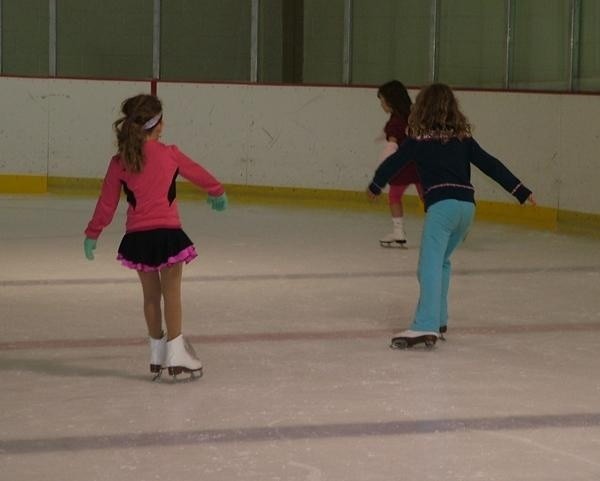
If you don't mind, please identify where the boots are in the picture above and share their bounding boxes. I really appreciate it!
[392,329,438,345]
[167,333,203,375]
[150,334,168,372]
[378,216,406,243]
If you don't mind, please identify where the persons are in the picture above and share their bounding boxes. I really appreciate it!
[83,94,229,377]
[374,79,425,244]
[365,83,538,351]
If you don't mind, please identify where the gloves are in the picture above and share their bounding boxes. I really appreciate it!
[84,237,97,259]
[206,192,228,210]
